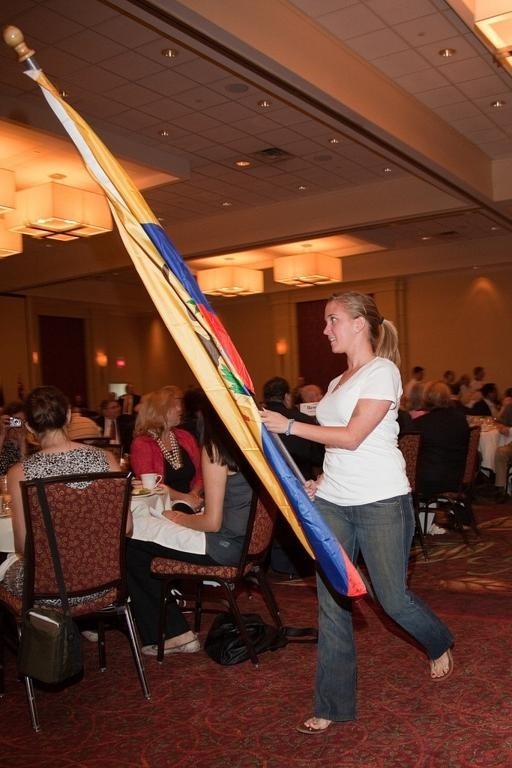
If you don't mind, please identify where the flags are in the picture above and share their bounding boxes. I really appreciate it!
[26,66,369,600]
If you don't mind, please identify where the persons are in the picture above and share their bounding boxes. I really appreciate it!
[256,288,454,736]
[396,364,512,545]
[1,376,326,655]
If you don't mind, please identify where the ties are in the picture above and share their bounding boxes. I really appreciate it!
[109,417,117,442]
[126,397,129,413]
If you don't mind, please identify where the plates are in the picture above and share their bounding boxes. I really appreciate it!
[131,489,156,498]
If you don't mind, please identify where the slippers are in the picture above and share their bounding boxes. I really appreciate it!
[296,716,336,735]
[428,649,454,682]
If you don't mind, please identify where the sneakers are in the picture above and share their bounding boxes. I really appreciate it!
[140,632,200,655]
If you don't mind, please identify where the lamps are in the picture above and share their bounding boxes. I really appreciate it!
[272,252,343,289]
[474,0,512,66]
[1,168,113,259]
[196,266,263,297]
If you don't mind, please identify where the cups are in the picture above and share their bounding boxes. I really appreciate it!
[140,472,163,490]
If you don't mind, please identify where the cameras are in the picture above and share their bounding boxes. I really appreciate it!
[9,416,22,429]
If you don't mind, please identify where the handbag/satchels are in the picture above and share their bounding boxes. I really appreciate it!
[270,545,313,576]
[204,612,289,665]
[17,607,84,692]
